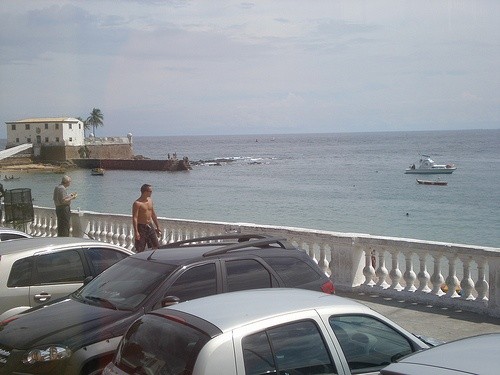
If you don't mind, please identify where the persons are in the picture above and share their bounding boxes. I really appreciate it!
[0,184,4,199]
[5,174,14,179]
[53,176,75,237]
[168,152,177,159]
[132,184,162,252]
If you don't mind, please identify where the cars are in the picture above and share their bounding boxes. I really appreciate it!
[97,286,443,375]
[0,236,136,324]
[376,331,500,375]
[0,229,34,243]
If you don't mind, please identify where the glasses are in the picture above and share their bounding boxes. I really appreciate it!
[147,191,152,192]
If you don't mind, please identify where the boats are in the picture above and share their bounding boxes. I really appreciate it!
[417,179,447,184]
[90,167,106,176]
[404,158,458,174]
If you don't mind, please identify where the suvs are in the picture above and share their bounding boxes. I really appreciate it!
[0,230,336,375]
